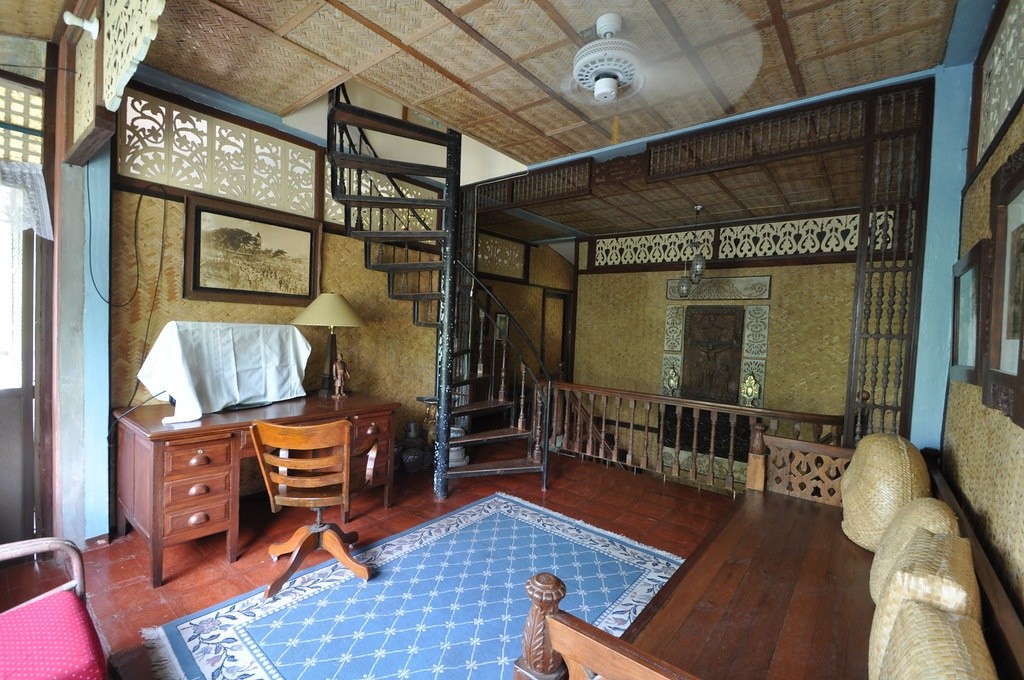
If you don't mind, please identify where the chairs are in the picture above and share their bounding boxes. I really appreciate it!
[0,538,110,680]
[248,418,370,599]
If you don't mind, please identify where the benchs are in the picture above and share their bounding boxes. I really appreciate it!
[512,422,1024,680]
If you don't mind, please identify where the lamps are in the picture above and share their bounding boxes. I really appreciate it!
[678,206,705,298]
[290,293,369,399]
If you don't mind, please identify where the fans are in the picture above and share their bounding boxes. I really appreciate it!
[464,0,766,144]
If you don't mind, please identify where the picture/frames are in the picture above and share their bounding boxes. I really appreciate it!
[983,142,1024,431]
[182,194,321,309]
[948,239,990,387]
[495,312,509,341]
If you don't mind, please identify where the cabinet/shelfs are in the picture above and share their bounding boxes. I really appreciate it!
[111,388,402,589]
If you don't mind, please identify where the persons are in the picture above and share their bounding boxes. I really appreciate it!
[333,353,350,396]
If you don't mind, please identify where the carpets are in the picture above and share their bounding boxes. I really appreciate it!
[138,492,686,680]
